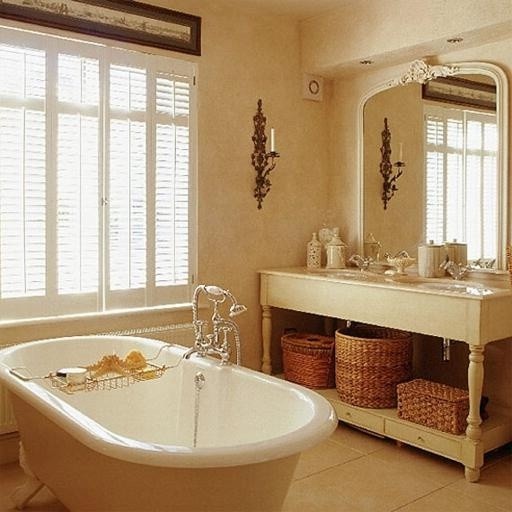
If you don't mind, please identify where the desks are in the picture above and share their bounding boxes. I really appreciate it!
[254,268,510,483]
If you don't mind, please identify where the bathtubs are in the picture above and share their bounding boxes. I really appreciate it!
[0,335,339,512]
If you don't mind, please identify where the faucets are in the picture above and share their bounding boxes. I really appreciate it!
[349,252,371,270]
[487,259,495,269]
[390,250,409,260]
[182,334,215,359]
[438,259,461,277]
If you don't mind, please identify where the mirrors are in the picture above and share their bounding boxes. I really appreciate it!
[354,57,507,273]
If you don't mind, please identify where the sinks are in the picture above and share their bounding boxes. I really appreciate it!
[309,269,372,279]
[391,279,485,288]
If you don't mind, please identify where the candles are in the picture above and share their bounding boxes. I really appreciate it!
[399,143,404,161]
[270,126,276,154]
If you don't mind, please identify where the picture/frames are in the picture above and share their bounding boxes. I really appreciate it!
[2,0,203,59]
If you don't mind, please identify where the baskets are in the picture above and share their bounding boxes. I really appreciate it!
[335,324,415,410]
[280,327,335,390]
[395,378,470,436]
